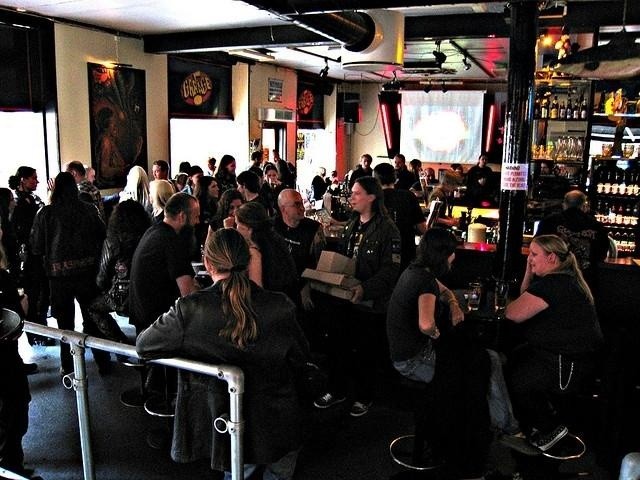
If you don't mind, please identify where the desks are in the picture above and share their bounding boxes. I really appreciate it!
[414,234,498,255]
[322,220,347,239]
[436,287,512,323]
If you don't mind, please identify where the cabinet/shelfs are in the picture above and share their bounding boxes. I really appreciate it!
[519,23,638,259]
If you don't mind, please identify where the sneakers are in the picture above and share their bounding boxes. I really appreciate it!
[313,392,347,408]
[349,400,373,416]
[532,424,568,452]
[498,433,542,455]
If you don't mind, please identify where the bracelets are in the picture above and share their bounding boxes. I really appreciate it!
[447,298,458,305]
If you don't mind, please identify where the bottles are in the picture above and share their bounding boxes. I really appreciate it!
[459,212,468,232]
[532,87,587,160]
[593,90,640,256]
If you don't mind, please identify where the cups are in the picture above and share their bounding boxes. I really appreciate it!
[468,283,481,311]
[495,280,508,310]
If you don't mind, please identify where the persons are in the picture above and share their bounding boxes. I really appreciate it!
[135,227,312,480]
[313,175,402,418]
[0,214,43,480]
[503,232,602,455]
[385,227,519,436]
[0,149,614,394]
